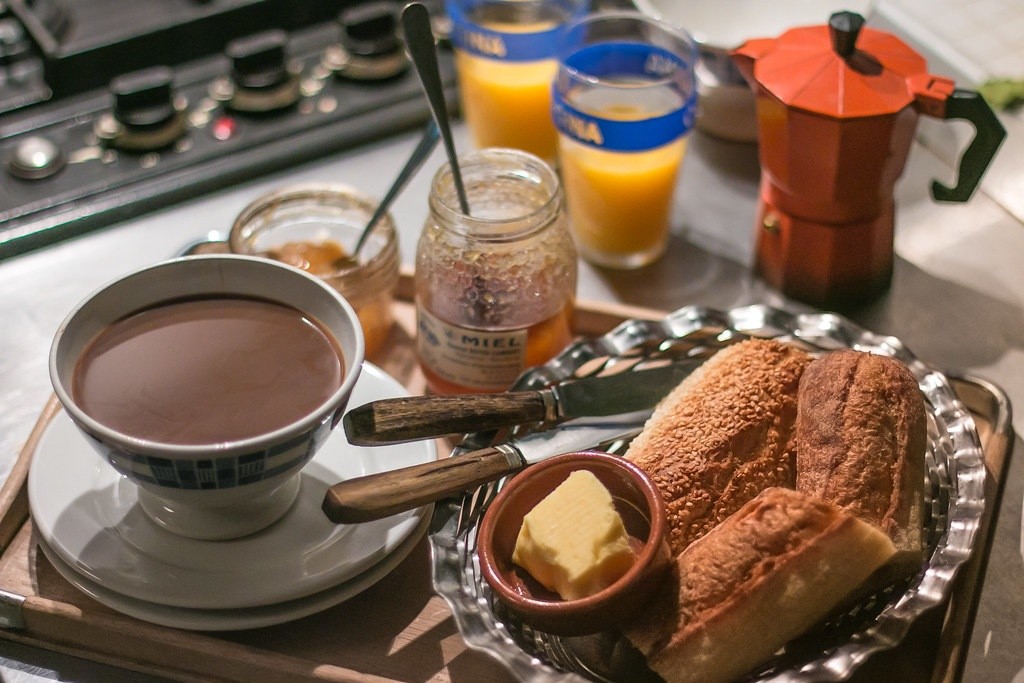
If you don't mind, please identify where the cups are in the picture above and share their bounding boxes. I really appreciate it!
[413,148,579,401]
[445,1,590,169]
[552,11,700,270]
[231,180,403,355]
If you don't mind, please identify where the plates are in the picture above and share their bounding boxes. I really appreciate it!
[432,300,985,683]
[26,364,440,635]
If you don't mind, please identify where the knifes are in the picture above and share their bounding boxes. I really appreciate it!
[323,355,701,526]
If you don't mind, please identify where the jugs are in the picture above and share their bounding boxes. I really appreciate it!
[729,9,1007,308]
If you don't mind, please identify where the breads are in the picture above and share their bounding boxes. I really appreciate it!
[613,338,928,683]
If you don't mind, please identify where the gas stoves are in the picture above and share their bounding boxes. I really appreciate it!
[0,2,678,263]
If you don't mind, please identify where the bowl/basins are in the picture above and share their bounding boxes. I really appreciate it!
[45,254,370,543]
[477,447,671,639]
